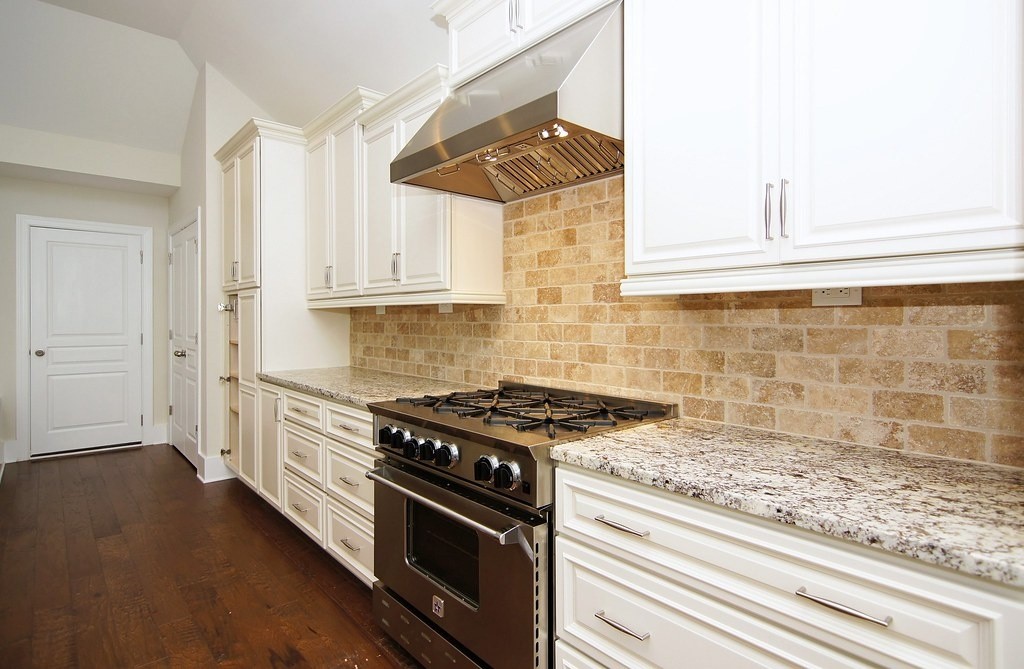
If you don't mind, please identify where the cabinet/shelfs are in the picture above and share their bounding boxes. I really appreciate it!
[257,381,377,589]
[552,464,1024,669]
[619,0,1024,297]
[221,135,259,492]
[304,63,507,308]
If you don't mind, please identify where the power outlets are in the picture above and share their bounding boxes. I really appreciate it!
[812,288,862,307]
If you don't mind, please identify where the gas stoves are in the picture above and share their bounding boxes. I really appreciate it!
[367,379,681,510]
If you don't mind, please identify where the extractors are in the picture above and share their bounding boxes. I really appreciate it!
[389,0,625,204]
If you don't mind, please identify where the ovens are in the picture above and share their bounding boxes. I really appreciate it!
[366,460,552,669]
